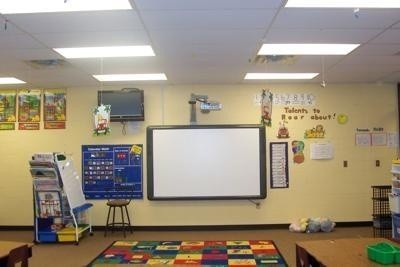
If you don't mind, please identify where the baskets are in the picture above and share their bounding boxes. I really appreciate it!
[367,242,400,264]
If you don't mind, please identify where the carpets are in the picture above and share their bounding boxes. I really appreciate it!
[85,238,290,267]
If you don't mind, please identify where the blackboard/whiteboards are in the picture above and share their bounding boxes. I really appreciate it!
[147,125,267,200]
[55,153,86,209]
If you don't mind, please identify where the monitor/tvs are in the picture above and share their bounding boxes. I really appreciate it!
[97,90,144,122]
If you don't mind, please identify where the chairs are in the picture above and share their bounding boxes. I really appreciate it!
[104,186,134,239]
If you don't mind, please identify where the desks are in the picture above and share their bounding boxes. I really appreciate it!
[295,236,400,267]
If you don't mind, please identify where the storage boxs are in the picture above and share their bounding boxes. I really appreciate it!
[385,189,400,236]
[38,225,83,242]
